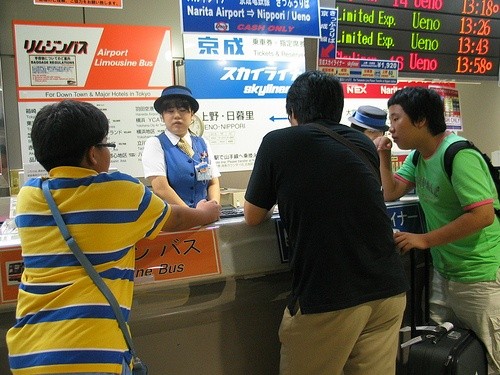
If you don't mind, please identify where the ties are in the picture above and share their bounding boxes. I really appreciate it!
[178,138,194,157]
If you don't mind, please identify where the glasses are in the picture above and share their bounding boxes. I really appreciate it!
[95,143,116,152]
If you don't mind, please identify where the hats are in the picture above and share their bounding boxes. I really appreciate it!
[153,85,199,114]
[348,106,390,131]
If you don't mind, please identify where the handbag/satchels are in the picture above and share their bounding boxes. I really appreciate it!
[132,364,149,375]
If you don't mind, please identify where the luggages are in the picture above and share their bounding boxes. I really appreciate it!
[407,246,489,375]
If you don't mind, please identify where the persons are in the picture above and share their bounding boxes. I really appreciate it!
[142,85,221,207]
[242,71,408,375]
[7,101,222,375]
[349,87,500,375]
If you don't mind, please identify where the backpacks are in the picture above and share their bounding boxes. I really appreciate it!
[412,140,500,220]
